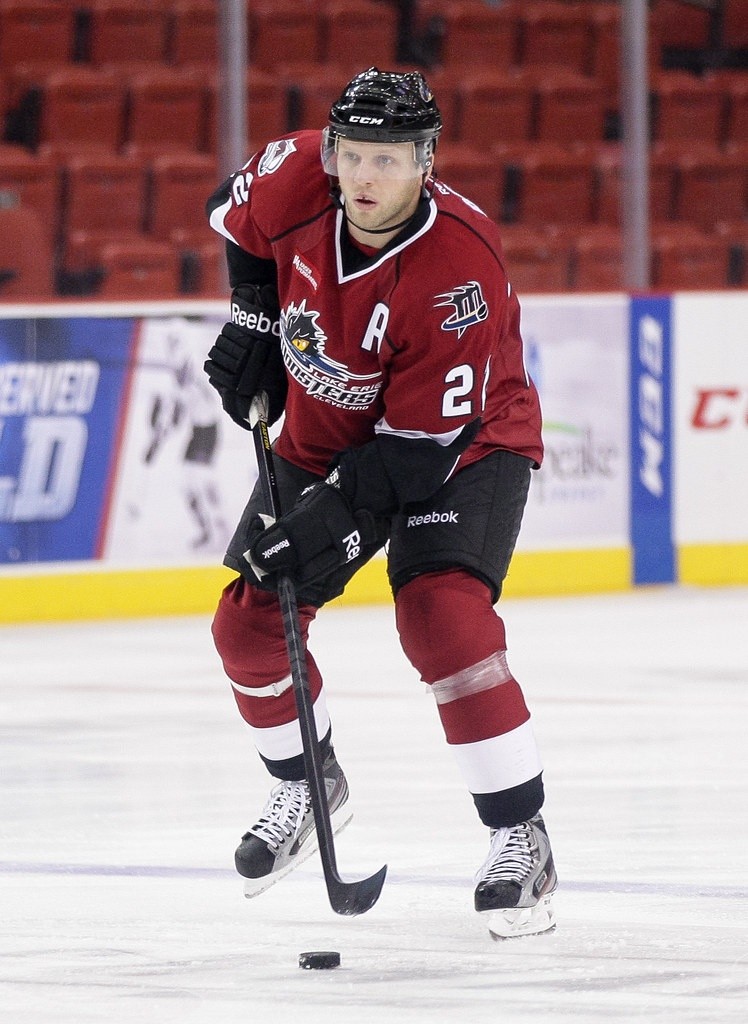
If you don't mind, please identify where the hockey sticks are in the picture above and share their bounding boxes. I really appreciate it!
[246,397,389,916]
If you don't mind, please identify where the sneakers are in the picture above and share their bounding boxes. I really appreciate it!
[235,743,354,899]
[473,812,560,941]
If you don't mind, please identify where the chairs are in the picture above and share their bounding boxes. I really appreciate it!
[0,0,748,299]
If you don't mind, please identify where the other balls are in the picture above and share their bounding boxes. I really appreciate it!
[299,948,341,971]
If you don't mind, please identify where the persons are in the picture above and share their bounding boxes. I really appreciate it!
[203,68,561,940]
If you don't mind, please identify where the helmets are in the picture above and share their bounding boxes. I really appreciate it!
[321,66,445,177]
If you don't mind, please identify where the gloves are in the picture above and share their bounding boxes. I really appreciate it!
[238,464,380,593]
[203,283,289,432]
